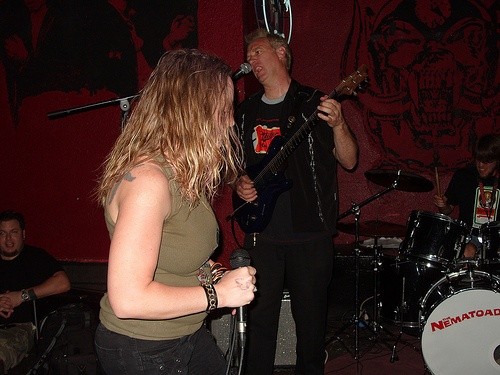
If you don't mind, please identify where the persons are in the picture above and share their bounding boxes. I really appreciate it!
[230,28,357,375]
[432,133,500,262]
[94,49,257,375]
[0,212,72,375]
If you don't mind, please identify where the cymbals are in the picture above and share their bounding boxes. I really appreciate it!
[363,167,434,193]
[336,219,406,240]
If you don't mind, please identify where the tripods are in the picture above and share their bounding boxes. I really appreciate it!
[325,176,416,363]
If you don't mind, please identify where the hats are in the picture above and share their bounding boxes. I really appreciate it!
[474,133,500,162]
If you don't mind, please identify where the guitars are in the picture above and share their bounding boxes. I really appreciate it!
[231,64,370,235]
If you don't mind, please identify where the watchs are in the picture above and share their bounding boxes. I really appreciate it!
[20,287,30,302]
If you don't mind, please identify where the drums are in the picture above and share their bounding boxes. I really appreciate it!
[400,209,469,268]
[417,270,500,375]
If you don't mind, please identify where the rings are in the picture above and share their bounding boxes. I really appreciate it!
[254,287,257,292]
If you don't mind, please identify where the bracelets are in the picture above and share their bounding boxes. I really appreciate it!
[28,286,37,300]
[209,262,227,286]
[201,282,218,313]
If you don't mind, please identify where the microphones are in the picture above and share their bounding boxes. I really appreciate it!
[229,248,251,347]
[230,62,252,82]
